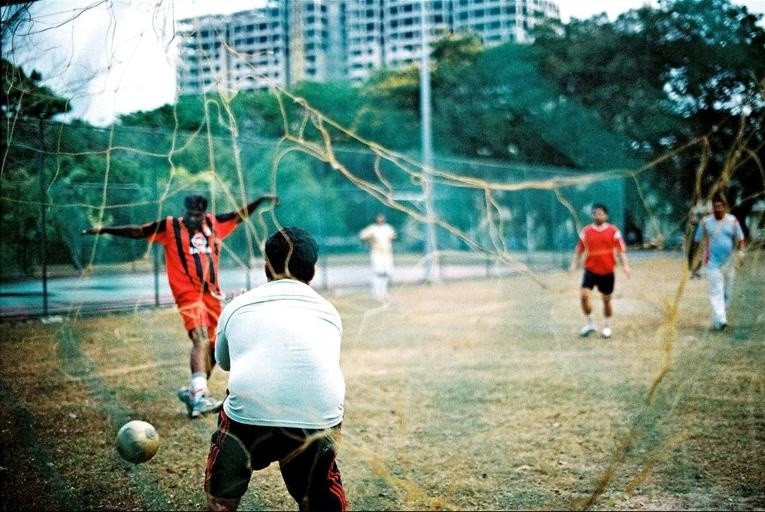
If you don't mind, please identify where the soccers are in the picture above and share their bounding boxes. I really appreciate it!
[115,420,162,464]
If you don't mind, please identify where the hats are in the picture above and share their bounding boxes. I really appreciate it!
[185,196,208,212]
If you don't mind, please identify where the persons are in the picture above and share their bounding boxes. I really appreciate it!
[357,210,398,302]
[687,195,749,333]
[80,193,280,421]
[203,226,349,511]
[565,201,633,339]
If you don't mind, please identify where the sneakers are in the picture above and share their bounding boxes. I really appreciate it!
[713,323,729,333]
[580,325,610,338]
[178,386,223,418]
